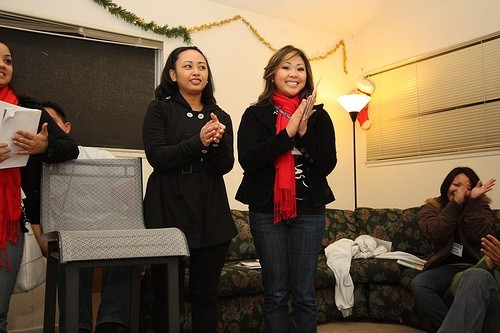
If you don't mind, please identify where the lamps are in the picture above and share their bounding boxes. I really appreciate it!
[336,93,371,208]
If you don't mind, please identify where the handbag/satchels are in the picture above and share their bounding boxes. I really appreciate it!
[11,187,52,294]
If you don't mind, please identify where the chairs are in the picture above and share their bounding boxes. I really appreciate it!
[41,157,190,333]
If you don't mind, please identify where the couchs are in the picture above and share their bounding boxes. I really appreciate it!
[182,208,500,332]
